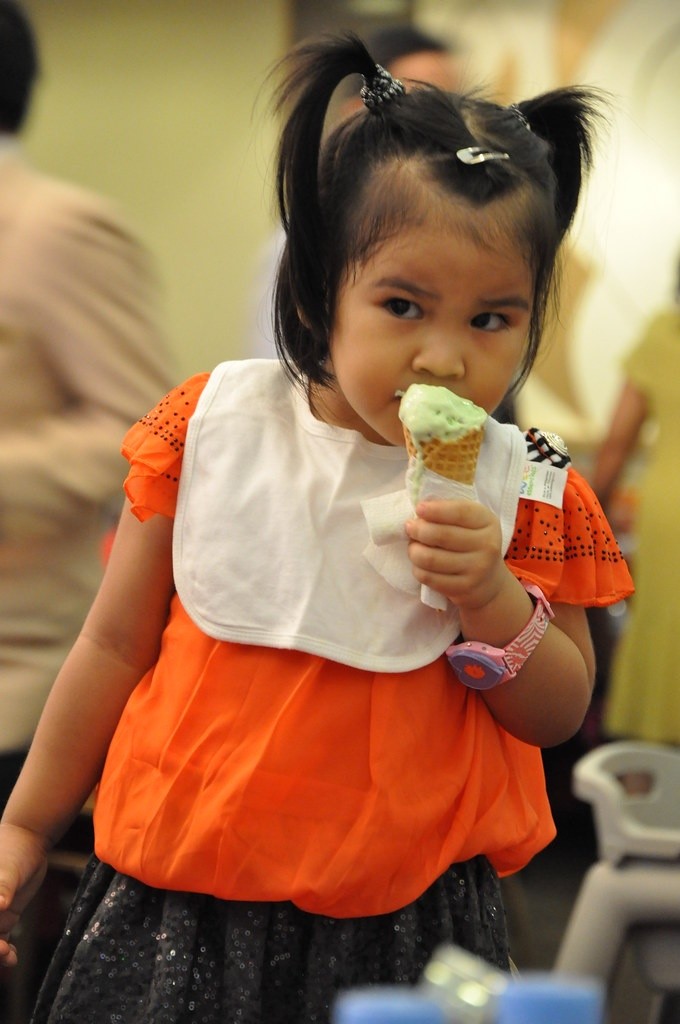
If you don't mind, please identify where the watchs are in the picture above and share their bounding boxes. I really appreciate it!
[445,578,555,690]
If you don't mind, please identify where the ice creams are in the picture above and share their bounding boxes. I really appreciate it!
[398,382,487,488]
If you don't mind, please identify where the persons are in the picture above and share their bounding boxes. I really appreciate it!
[0,31,635,1024]
[253,25,457,362]
[587,302,680,794]
[0,1,173,817]
